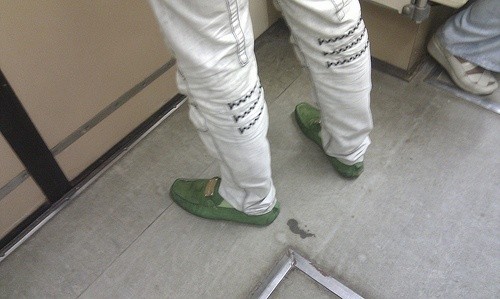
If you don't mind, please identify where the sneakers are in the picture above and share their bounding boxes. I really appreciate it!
[427,31,498,95]
[169,176,281,226]
[295,102,364,177]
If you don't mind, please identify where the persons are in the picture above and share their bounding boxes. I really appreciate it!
[149,0,373,226]
[427,0,500,96]
